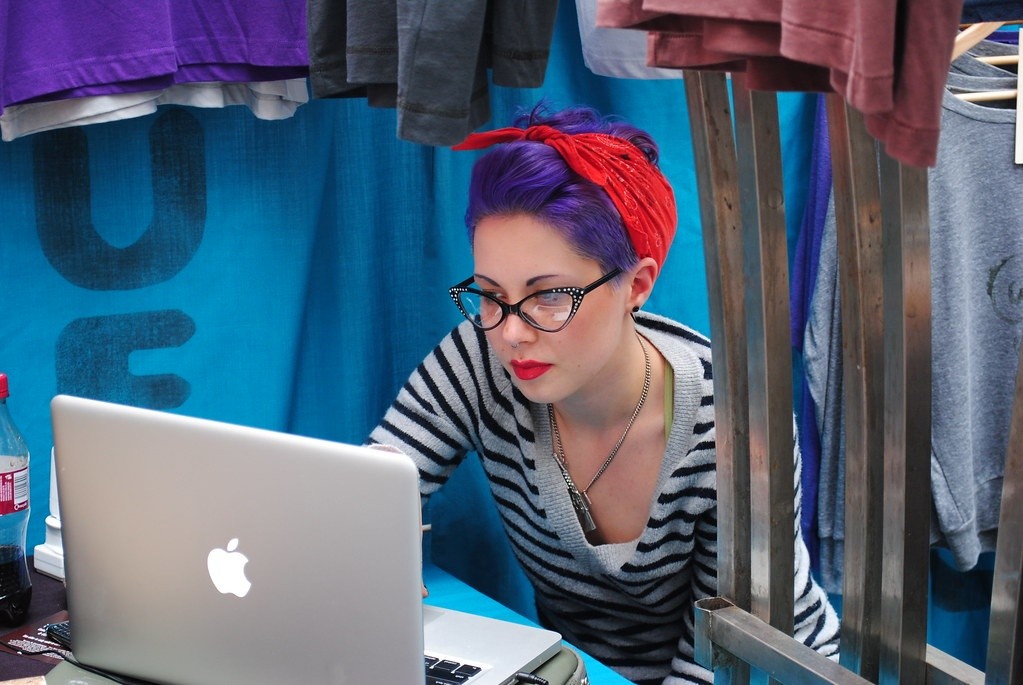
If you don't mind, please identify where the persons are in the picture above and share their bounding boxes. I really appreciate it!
[362,97,842,685]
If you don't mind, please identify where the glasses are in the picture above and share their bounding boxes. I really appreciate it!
[449,266,623,332]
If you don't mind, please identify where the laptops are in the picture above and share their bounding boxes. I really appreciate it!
[50,395,562,685]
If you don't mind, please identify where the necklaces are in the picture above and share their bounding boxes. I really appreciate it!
[547,332,651,533]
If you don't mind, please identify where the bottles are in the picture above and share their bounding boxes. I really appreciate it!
[0,373,32,628]
